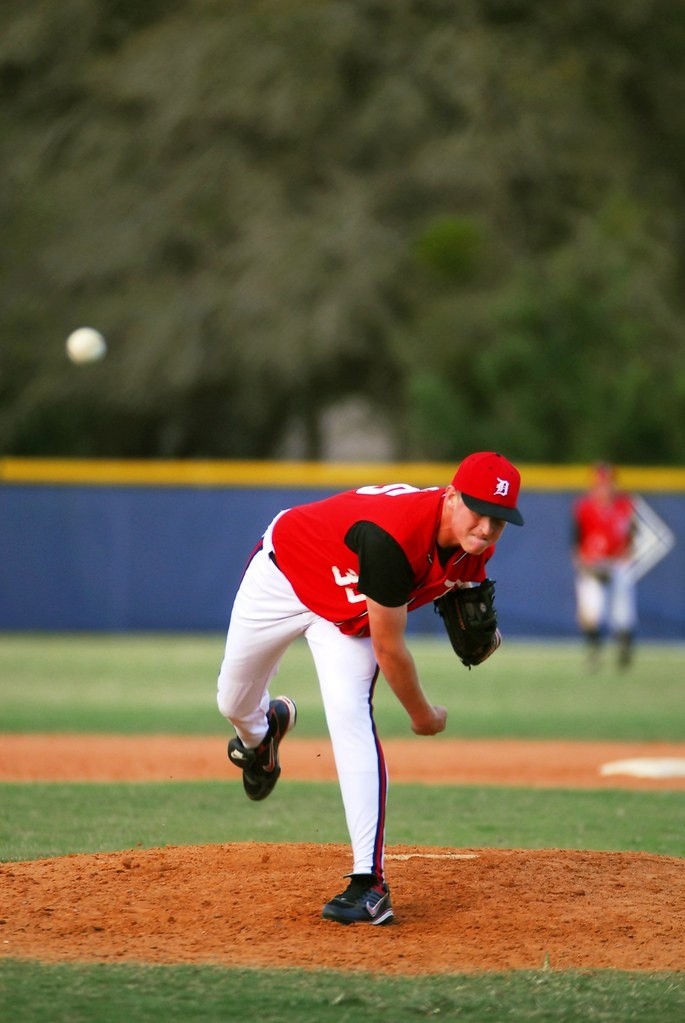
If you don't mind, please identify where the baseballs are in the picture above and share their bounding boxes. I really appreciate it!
[65,326,107,369]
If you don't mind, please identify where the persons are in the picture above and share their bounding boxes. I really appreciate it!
[217,452,524,925]
[568,449,639,675]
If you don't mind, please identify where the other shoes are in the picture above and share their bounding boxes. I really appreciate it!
[612,627,633,668]
[580,626,603,657]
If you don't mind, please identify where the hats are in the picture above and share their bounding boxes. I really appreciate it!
[451,450,524,527]
[589,462,617,485]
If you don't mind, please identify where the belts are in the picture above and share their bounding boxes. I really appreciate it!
[262,537,280,571]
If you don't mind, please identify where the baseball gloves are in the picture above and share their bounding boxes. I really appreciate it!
[433,578,502,667]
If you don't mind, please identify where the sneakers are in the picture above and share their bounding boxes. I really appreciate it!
[228,694,297,802]
[321,873,393,926]
